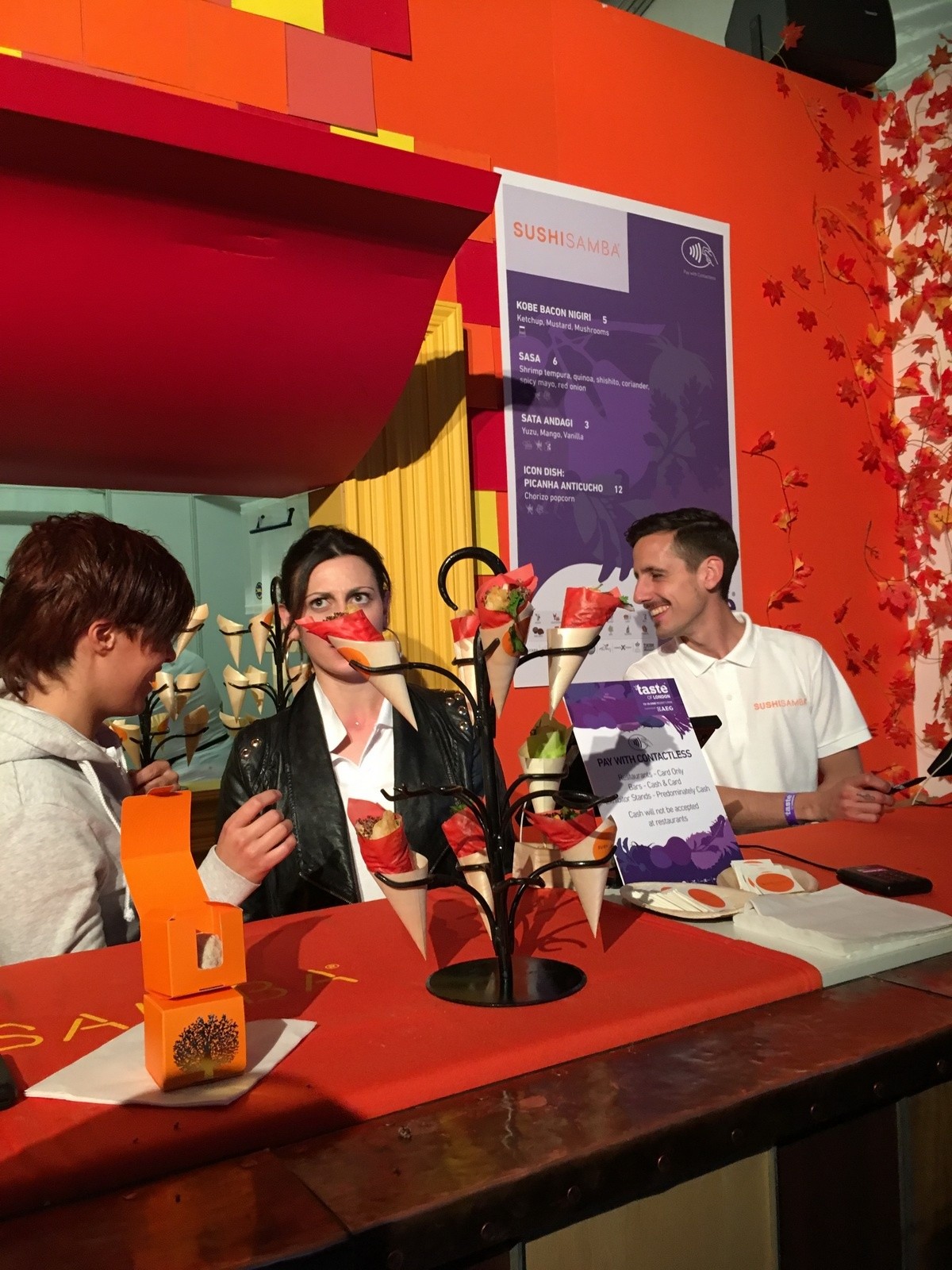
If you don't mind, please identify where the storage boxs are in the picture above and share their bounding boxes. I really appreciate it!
[116,790,249,1091]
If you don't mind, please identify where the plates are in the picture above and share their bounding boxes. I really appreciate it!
[718,867,819,895]
[619,882,757,918]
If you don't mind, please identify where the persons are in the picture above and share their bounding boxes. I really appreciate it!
[626,508,894,836]
[214,521,516,923]
[0,508,296,967]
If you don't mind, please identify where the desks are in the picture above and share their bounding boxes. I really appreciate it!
[5,793,951,1269]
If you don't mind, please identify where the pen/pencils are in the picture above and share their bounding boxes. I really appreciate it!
[888,776,927,794]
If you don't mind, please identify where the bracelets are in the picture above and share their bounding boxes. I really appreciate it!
[784,793,799,826]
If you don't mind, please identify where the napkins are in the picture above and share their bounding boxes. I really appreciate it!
[733,884,952,963]
[22,1014,319,1110]
